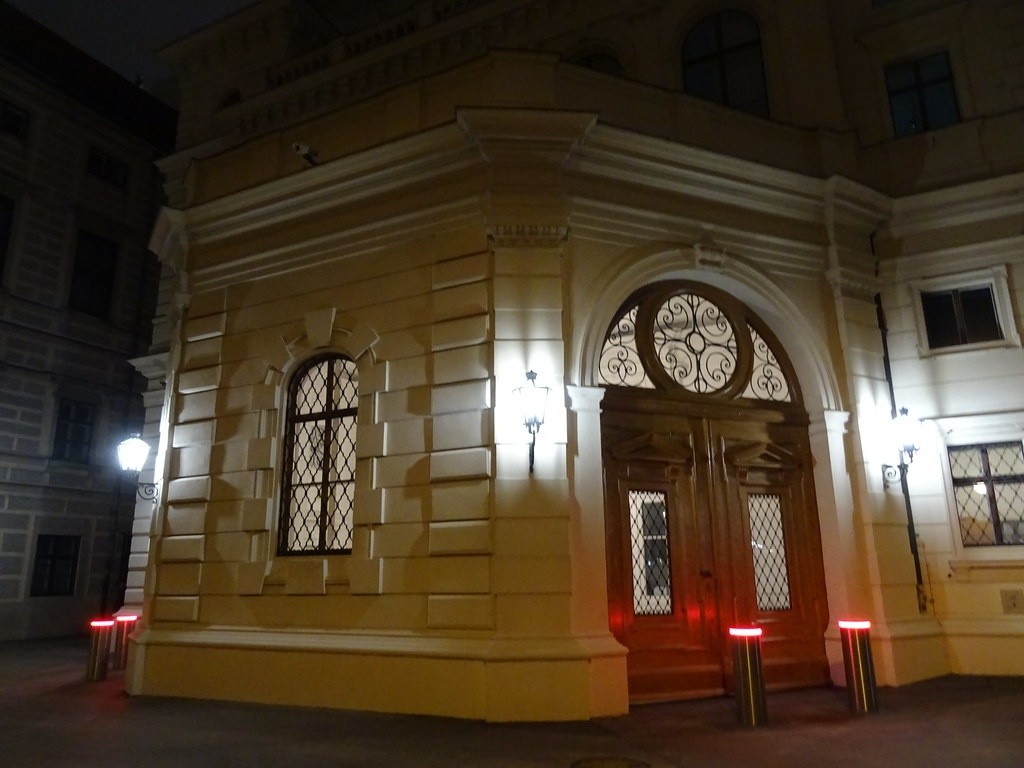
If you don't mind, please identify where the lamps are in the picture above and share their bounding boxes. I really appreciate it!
[116,432,159,505]
[511,370,549,469]
[882,406,923,491]
